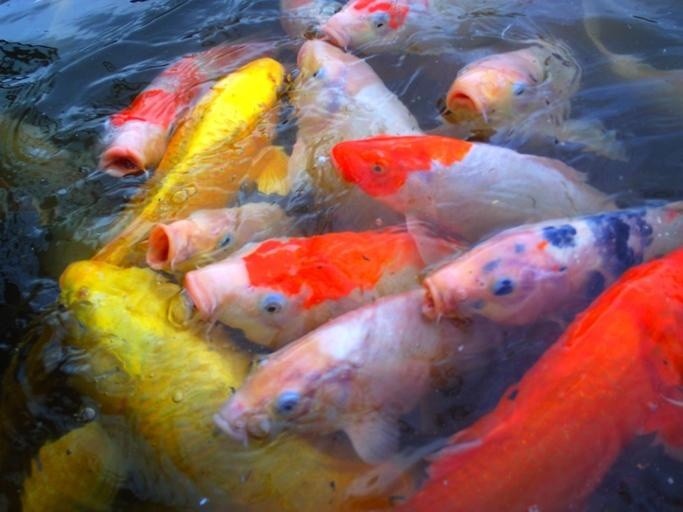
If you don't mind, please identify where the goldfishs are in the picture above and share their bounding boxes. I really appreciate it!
[0,0,683,512]
